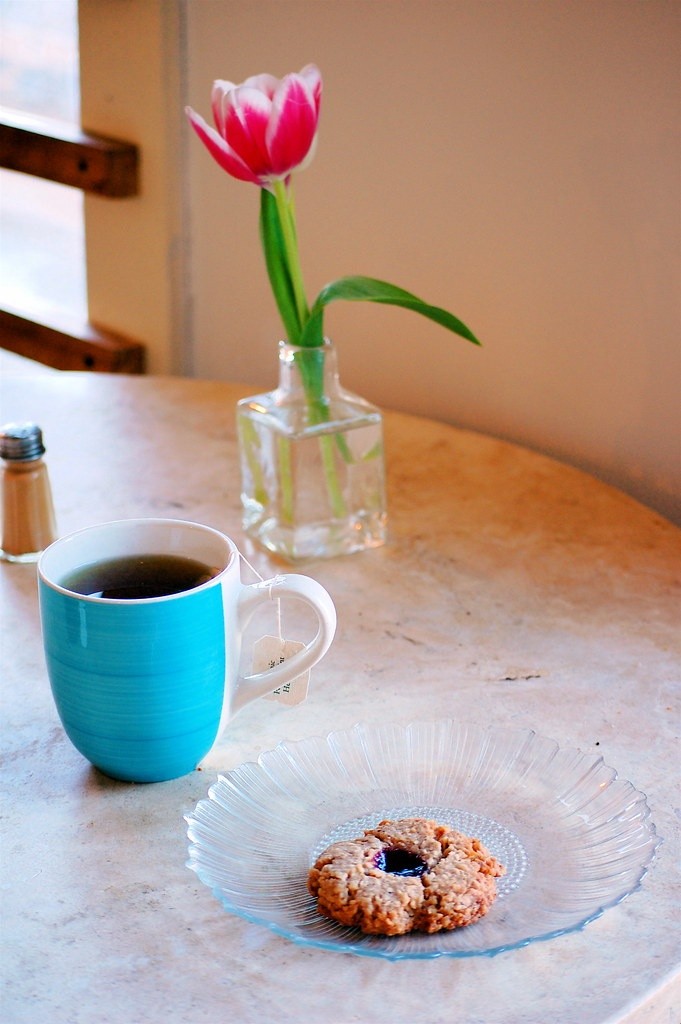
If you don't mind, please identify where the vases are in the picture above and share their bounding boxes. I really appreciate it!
[236,339,387,565]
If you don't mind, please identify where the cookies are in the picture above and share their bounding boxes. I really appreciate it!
[306,818,504,937]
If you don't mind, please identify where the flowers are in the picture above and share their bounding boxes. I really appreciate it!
[182,61,482,346]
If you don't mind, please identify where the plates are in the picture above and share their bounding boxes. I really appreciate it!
[184,716,663,962]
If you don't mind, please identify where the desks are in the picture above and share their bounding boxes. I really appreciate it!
[0,371,681,1024]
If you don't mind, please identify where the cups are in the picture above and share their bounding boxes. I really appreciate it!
[37,519,336,783]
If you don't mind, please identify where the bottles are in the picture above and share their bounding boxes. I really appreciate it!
[235,342,388,559]
[0,423,59,564]
